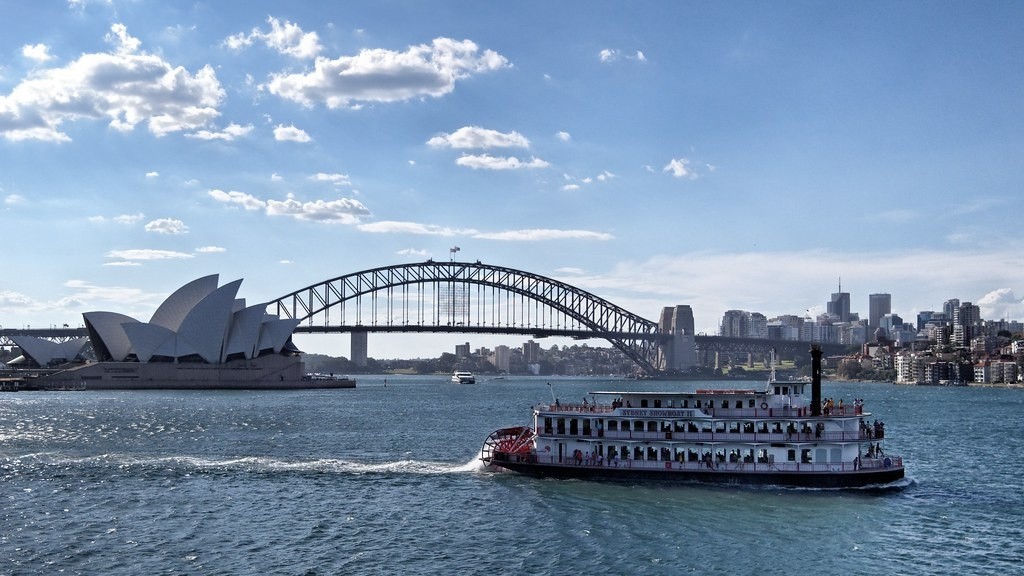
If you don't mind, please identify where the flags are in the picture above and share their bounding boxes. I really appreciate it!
[450,247,460,252]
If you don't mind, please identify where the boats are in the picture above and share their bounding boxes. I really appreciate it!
[479,343,906,490]
[452,371,475,384]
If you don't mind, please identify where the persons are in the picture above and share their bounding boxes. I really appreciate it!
[581,397,588,412]
[626,400,633,407]
[862,420,885,440]
[853,398,864,416]
[869,443,882,459]
[823,398,834,415]
[544,417,824,469]
[853,457,858,471]
[839,399,844,415]
[555,398,563,412]
[612,398,623,411]
[592,396,597,411]
[704,403,709,415]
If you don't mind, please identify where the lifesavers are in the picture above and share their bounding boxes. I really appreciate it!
[761,402,769,410]
[665,462,671,468]
